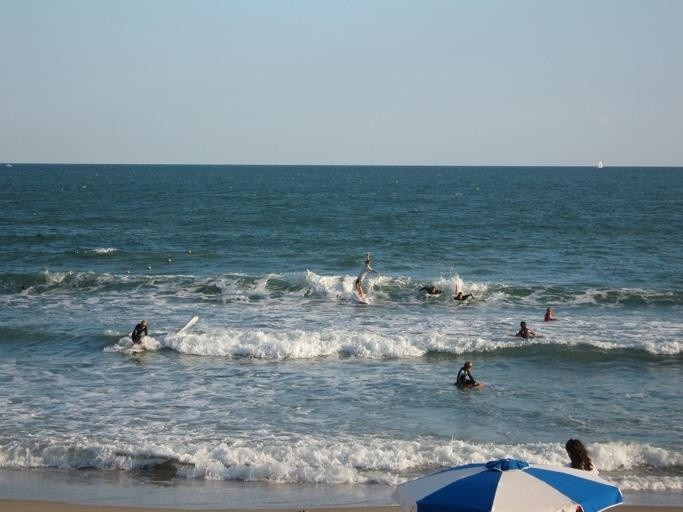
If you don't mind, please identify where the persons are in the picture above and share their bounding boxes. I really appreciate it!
[131,319,147,344]
[516,321,542,338]
[355,251,378,300]
[544,306,557,321]
[418,286,442,295]
[454,291,475,300]
[563,438,599,475]
[455,360,477,387]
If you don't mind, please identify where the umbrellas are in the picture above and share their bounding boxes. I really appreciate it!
[391,458,626,512]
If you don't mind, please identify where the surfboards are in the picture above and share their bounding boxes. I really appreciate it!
[455,383,482,388]
[176,315,199,335]
[352,291,368,304]
